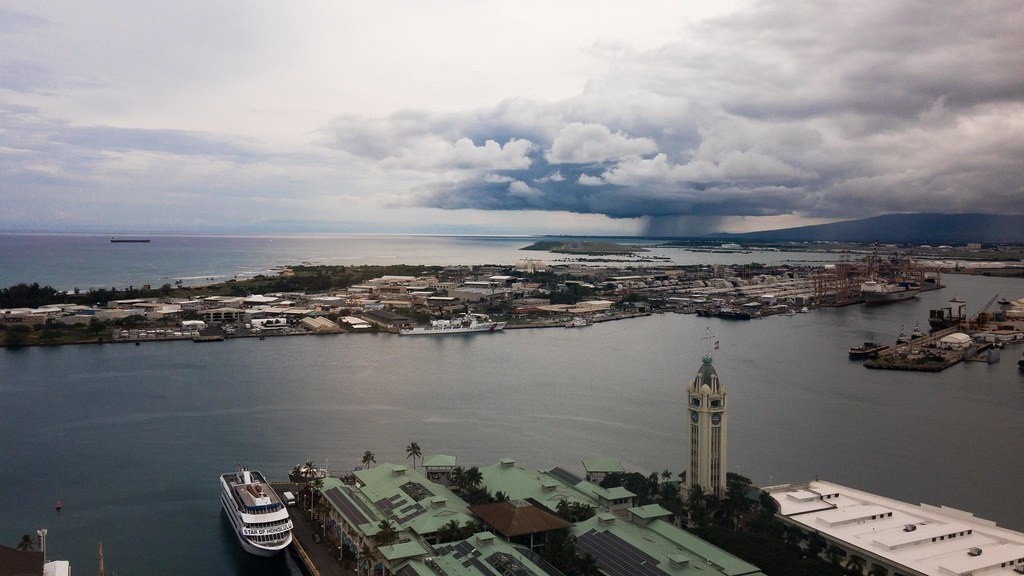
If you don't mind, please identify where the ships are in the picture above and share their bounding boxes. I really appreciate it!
[218,463,293,558]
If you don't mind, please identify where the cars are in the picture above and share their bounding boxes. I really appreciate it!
[312,534,322,544]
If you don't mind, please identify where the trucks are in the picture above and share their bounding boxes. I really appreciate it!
[284,492,297,508]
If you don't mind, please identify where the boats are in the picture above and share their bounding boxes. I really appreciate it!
[911,319,927,340]
[928,297,967,331]
[564,315,588,328]
[720,308,750,321]
[861,280,924,304]
[399,310,508,335]
[800,307,810,313]
[785,309,796,316]
[695,299,722,317]
[847,338,890,361]
[896,323,910,345]
